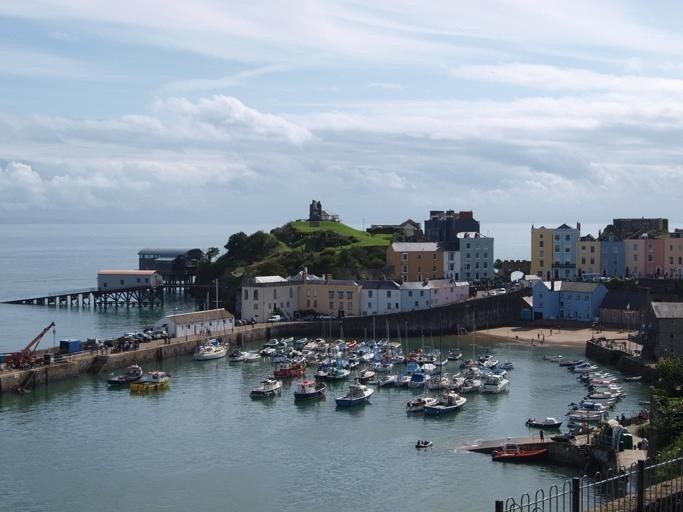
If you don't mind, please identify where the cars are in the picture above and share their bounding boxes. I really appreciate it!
[118,330,166,345]
[486,290,497,296]
[498,287,507,294]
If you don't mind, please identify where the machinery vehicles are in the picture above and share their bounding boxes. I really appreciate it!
[5,321,55,369]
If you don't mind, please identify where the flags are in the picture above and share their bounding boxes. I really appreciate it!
[625,302,629,311]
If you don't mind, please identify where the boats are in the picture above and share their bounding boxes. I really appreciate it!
[525,416,563,429]
[541,353,625,442]
[490,436,548,463]
[415,439,433,448]
[334,383,375,409]
[423,391,468,416]
[406,395,437,413]
[128,370,172,392]
[623,374,643,382]
[250,378,284,400]
[193,339,230,362]
[107,365,144,383]
[230,316,514,395]
[294,379,327,400]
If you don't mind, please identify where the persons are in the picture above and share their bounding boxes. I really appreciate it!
[539,428,545,443]
[250,317,256,328]
[528,325,560,348]
[416,439,430,446]
